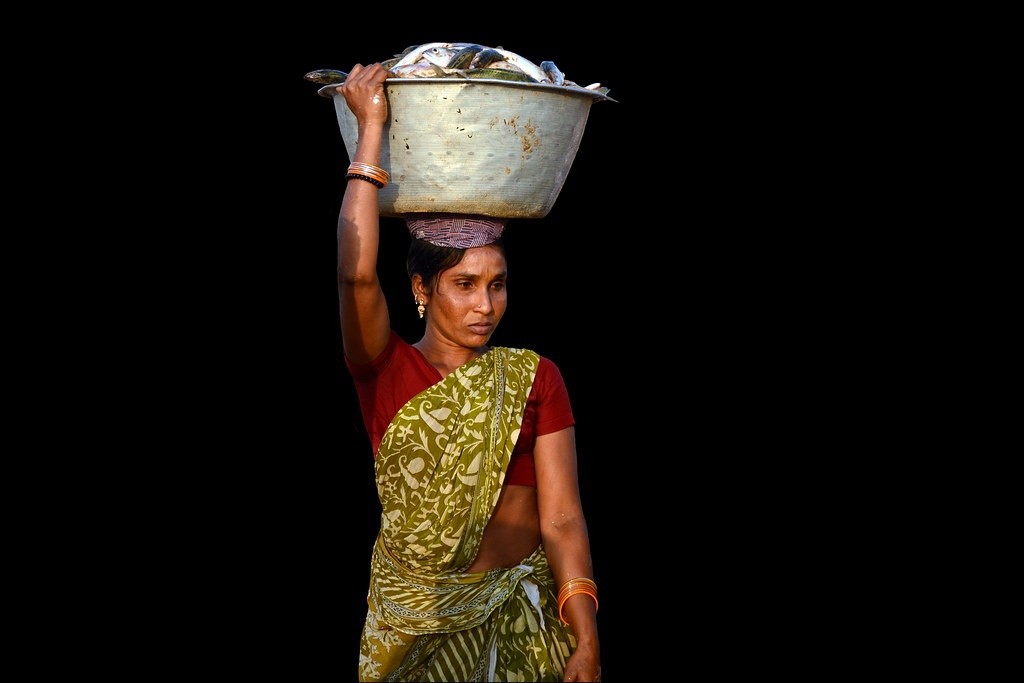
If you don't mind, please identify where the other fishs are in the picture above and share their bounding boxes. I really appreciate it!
[381,42,610,95]
[304,69,348,84]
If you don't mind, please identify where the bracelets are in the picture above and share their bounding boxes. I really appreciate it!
[557,577,599,625]
[344,161,389,189]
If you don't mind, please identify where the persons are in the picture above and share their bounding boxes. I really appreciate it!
[336,62,600,683]
